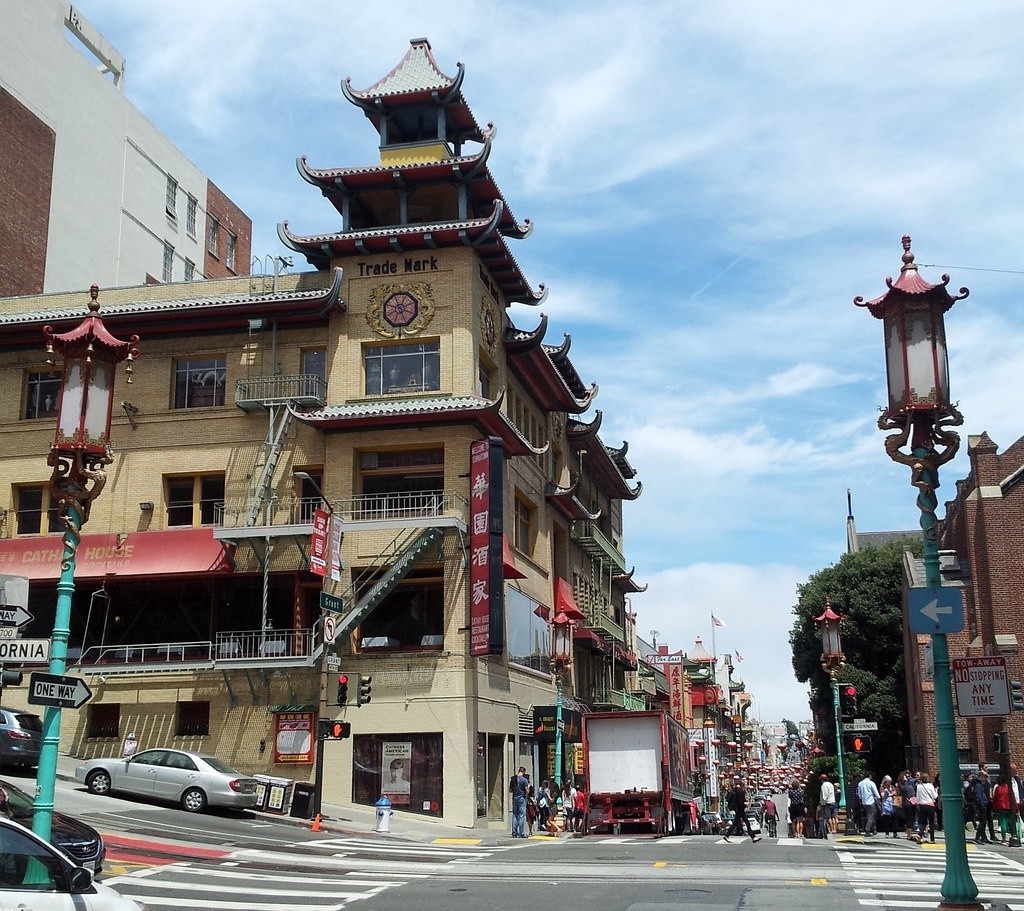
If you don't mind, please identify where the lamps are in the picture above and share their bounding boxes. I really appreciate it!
[98,677,106,684]
[139,501,154,510]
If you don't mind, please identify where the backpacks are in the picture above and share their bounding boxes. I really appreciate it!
[725,789,738,809]
[965,782,976,802]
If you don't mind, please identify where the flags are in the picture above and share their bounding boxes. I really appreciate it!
[735,650,744,663]
[712,614,725,627]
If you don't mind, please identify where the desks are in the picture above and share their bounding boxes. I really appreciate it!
[361,637,400,648]
[421,635,444,646]
[67,649,81,659]
[219,642,242,653]
[388,383,432,393]
[114,650,141,658]
[259,641,285,653]
[156,644,182,656]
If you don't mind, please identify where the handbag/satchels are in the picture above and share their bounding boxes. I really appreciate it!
[1016,814,1024,839]
[893,795,903,807]
[910,796,917,805]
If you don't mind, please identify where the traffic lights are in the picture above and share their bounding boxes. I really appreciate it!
[356,674,375,708]
[0,668,23,688]
[851,735,872,753]
[337,674,348,706]
[331,721,351,740]
[845,684,858,718]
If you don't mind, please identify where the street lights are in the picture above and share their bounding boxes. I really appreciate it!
[293,468,334,821]
[16,281,144,893]
[811,594,865,838]
[851,229,988,911]
[545,602,578,813]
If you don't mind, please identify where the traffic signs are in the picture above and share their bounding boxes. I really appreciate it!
[0,637,49,664]
[0,604,36,629]
[25,669,94,711]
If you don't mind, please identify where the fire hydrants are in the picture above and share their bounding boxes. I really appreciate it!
[375,795,396,833]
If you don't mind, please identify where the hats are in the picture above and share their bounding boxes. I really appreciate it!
[819,774,828,779]
[766,796,771,800]
[980,770,989,777]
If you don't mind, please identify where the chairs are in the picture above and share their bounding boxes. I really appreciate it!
[172,759,180,767]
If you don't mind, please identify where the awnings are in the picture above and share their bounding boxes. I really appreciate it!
[573,628,638,671]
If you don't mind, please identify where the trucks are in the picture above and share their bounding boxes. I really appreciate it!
[581,706,700,836]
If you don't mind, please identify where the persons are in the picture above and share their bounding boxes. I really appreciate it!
[762,795,779,828]
[789,774,842,839]
[963,762,1024,845]
[510,767,584,838]
[723,782,762,844]
[383,759,410,791]
[847,770,943,843]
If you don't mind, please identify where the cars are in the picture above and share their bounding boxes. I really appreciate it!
[0,706,43,774]
[71,746,260,813]
[703,789,772,835]
[0,779,107,878]
[0,814,147,911]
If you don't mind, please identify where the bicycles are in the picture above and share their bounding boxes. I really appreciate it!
[764,818,779,839]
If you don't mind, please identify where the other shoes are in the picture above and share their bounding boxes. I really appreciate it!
[752,836,761,843]
[793,827,936,843]
[973,835,1009,845]
[512,823,583,838]
[723,837,734,843]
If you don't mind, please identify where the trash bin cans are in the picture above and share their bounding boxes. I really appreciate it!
[265,777,294,815]
[290,782,314,819]
[248,775,272,812]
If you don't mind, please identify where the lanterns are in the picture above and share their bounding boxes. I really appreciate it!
[696,737,809,791]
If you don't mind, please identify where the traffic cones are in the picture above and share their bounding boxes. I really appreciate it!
[310,813,321,832]
